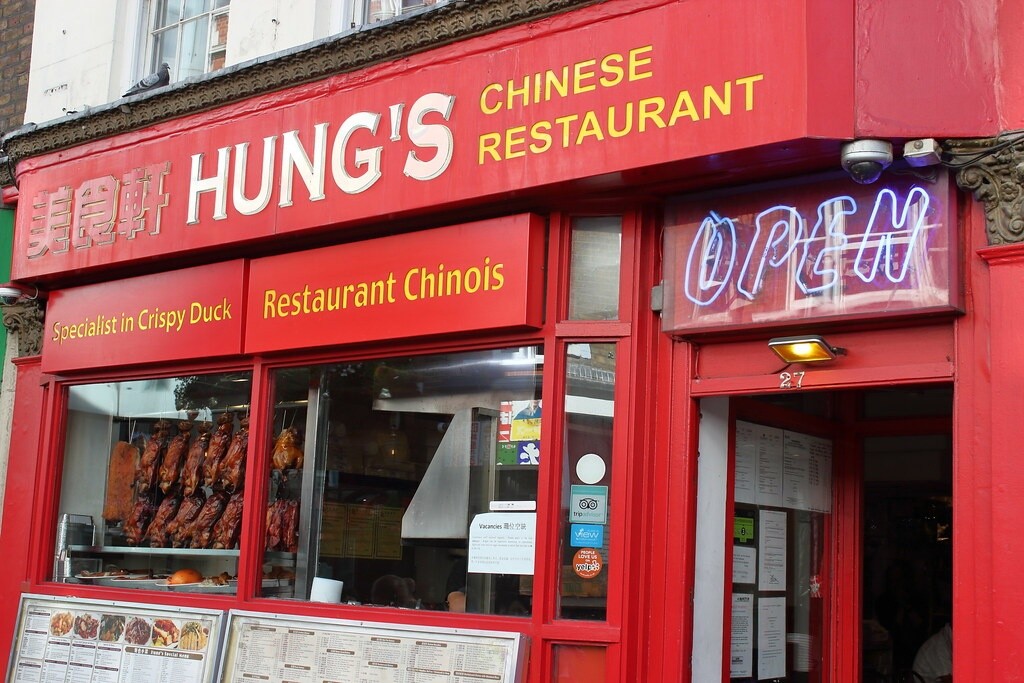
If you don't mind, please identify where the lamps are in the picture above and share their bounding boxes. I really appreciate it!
[767,334,848,374]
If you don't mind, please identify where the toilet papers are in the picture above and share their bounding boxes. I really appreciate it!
[309,576,343,604]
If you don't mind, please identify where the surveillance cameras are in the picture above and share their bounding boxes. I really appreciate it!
[841,138,893,184]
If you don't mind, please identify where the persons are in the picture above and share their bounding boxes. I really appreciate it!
[912,605,952,683]
[445,557,496,615]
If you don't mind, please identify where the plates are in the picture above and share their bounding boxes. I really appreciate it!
[49,609,208,654]
[74,569,295,594]
